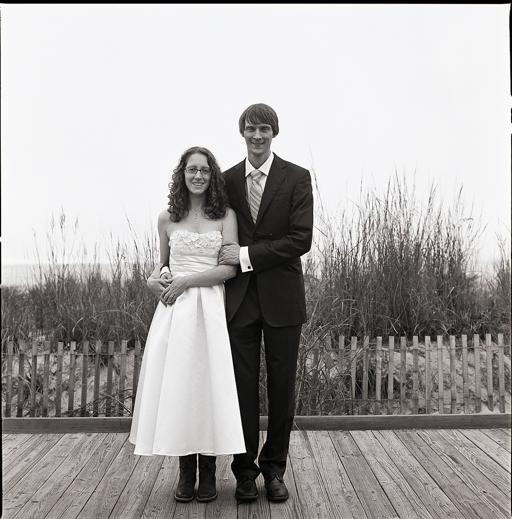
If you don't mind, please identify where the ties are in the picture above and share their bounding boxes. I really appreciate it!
[250,169,262,224]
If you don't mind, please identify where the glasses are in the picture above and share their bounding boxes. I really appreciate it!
[185,166,210,174]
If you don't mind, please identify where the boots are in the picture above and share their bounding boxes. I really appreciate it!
[196,453,217,502]
[175,454,197,502]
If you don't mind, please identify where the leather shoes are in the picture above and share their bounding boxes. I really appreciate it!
[235,478,258,499]
[265,476,288,500]
